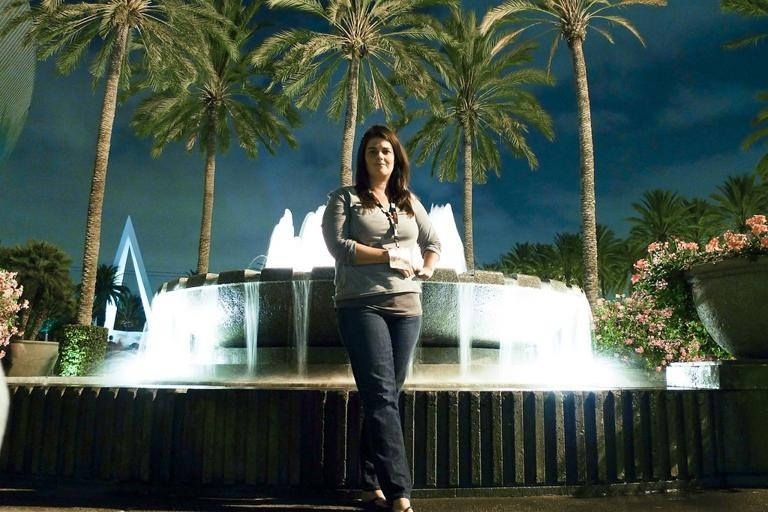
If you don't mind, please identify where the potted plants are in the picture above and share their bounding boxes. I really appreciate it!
[0,239,79,376]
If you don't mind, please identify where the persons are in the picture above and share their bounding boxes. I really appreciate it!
[322,126,442,512]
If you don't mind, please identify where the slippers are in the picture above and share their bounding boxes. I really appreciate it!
[359,495,391,509]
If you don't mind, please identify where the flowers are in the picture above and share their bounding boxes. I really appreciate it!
[588,214,768,380]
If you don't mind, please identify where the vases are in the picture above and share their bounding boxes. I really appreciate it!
[680,254,767,364]
